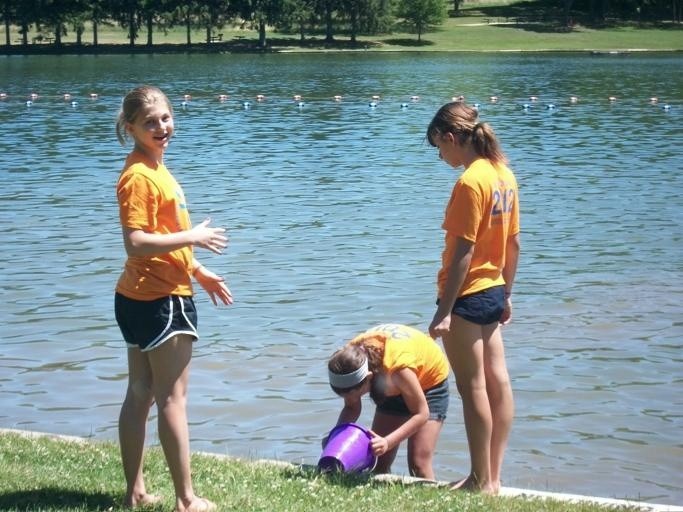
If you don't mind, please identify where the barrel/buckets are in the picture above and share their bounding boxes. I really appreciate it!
[317,422,378,476]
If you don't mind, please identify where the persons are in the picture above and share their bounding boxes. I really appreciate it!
[115,86,232,512]
[322,324,451,477]
[428,102,522,494]
